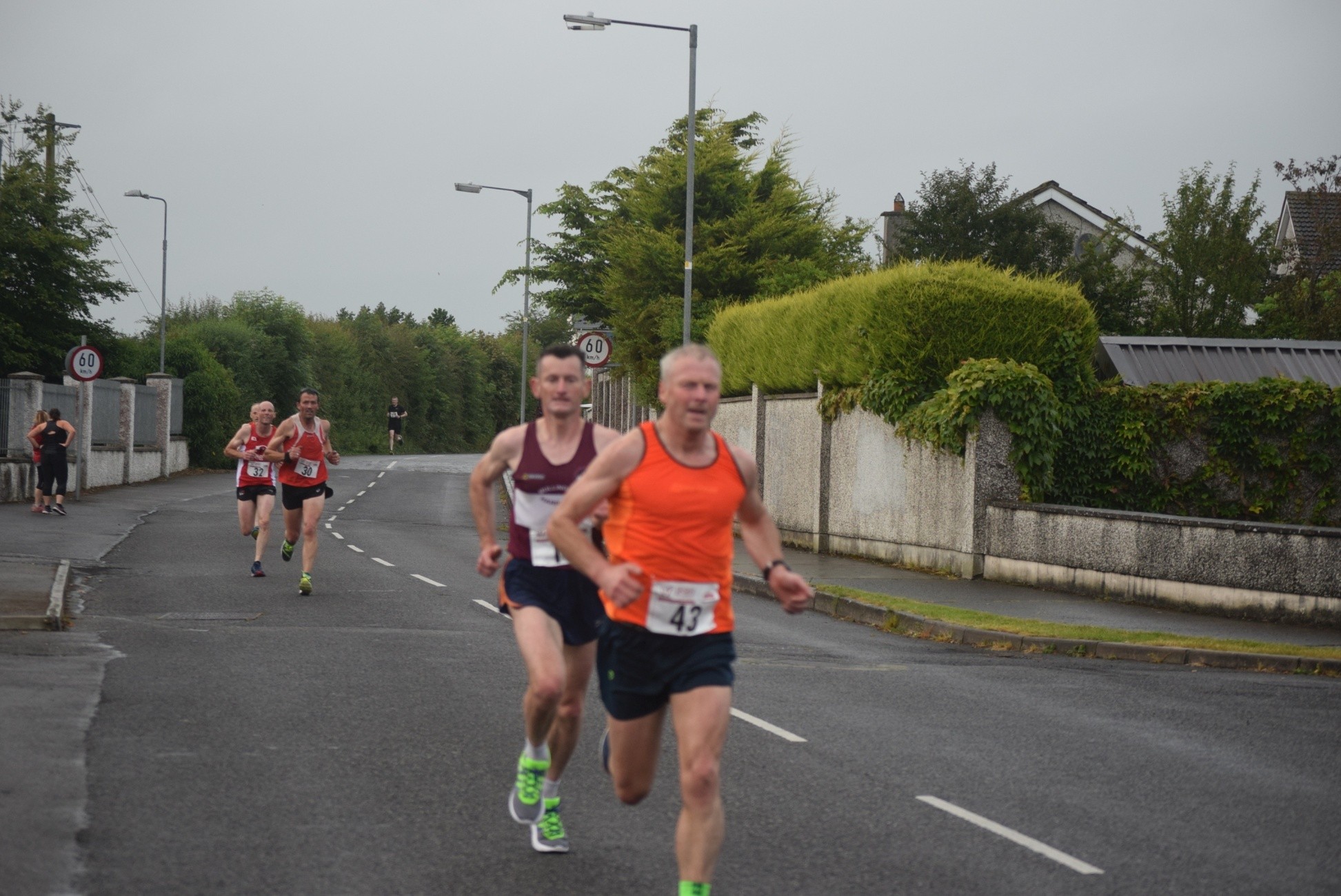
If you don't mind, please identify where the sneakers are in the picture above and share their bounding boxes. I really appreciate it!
[508,746,552,824]
[531,797,569,853]
[281,540,295,561]
[299,572,312,596]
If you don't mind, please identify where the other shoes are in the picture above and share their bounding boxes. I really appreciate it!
[42,507,53,514]
[600,729,610,774]
[252,526,259,539]
[389,450,394,455]
[250,564,266,577]
[32,505,45,513]
[397,435,403,446]
[52,504,67,515]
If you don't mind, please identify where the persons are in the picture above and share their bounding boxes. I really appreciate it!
[27,408,76,516]
[469,342,627,854]
[223,399,284,577]
[546,344,814,896]
[261,388,339,596]
[386,396,408,455]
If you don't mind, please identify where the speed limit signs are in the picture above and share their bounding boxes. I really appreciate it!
[68,345,103,381]
[577,331,611,368]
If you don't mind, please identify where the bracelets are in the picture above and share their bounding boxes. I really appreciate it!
[284,450,293,464]
[399,415,401,419]
[37,444,40,449]
[762,558,790,581]
[326,452,331,460]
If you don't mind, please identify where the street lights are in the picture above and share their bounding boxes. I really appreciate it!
[122,188,168,373]
[564,13,699,354]
[454,182,533,426]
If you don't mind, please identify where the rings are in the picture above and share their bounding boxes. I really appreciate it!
[296,453,298,455]
